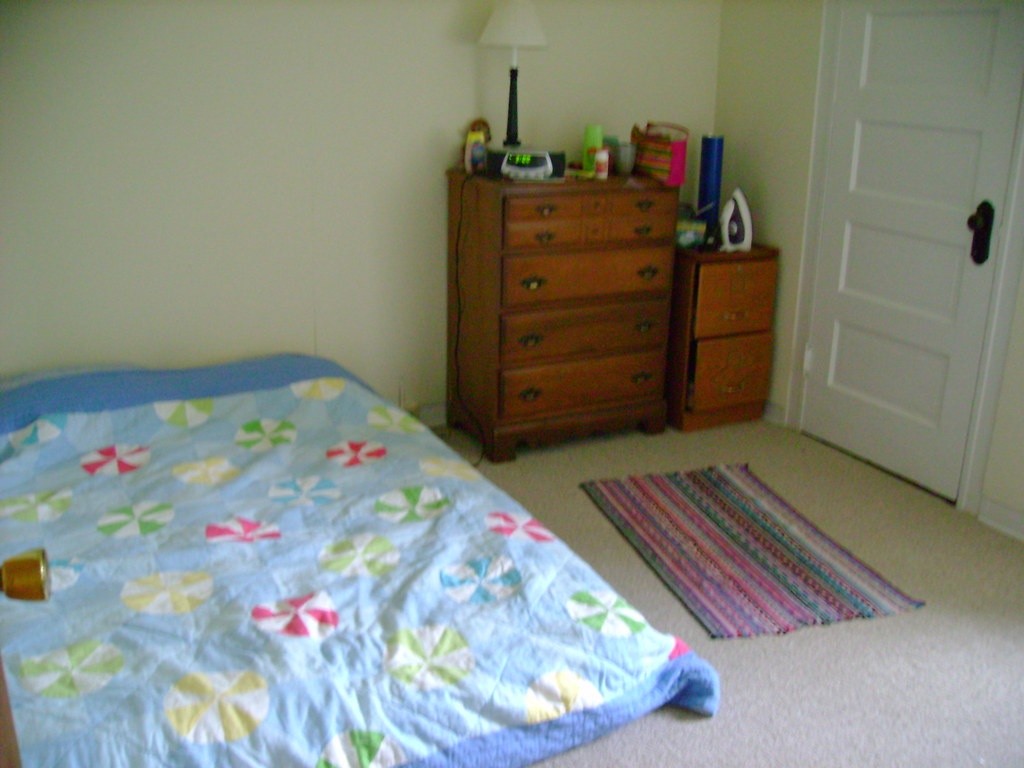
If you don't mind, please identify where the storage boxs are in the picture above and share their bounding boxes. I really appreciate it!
[678,211,708,248]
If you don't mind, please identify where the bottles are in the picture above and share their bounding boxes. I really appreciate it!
[593,149,609,180]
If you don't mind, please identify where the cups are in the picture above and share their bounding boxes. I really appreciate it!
[0,550,51,601]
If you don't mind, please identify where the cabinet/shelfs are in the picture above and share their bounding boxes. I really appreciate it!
[445,166,679,462]
[666,239,780,432]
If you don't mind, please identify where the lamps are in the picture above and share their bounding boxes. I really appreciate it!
[479,0,548,146]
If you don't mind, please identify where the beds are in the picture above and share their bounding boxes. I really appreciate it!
[0,348,723,768]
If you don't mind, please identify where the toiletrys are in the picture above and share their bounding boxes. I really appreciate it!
[568,123,635,179]
[464,128,486,176]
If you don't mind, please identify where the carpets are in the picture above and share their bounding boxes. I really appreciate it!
[580,463,927,642]
